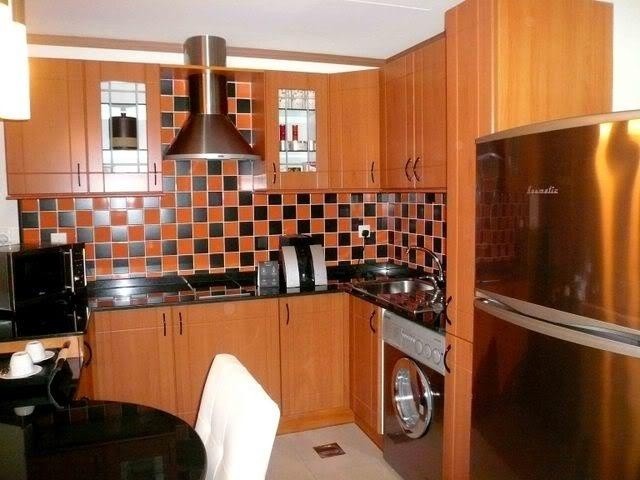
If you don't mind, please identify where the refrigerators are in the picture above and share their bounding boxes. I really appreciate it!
[463,106,639,480]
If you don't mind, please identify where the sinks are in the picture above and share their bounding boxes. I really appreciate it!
[357,279,437,296]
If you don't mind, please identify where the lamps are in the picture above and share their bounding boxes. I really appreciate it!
[0,19,31,122]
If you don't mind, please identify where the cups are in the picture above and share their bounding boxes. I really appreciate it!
[24,341,47,360]
[9,350,34,375]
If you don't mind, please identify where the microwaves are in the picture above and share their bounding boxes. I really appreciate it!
[0,239,87,313]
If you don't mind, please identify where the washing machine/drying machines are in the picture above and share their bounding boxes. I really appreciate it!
[381,308,446,480]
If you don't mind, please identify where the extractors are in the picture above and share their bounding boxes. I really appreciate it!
[161,36,264,164]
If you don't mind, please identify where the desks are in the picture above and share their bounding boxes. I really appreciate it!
[0,354,208,479]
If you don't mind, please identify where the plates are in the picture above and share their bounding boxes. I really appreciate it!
[0,364,44,380]
[32,350,56,364]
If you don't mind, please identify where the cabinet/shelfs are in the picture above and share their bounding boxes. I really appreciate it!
[279,292,383,449]
[254,68,380,195]
[380,30,446,193]
[6,57,165,200]
[442,0,612,480]
[76,298,279,429]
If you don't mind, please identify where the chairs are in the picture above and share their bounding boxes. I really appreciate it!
[193,352,279,480]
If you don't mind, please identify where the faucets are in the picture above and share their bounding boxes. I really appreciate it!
[428,287,445,327]
[404,246,445,282]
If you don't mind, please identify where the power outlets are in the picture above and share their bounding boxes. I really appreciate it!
[358,224,370,238]
